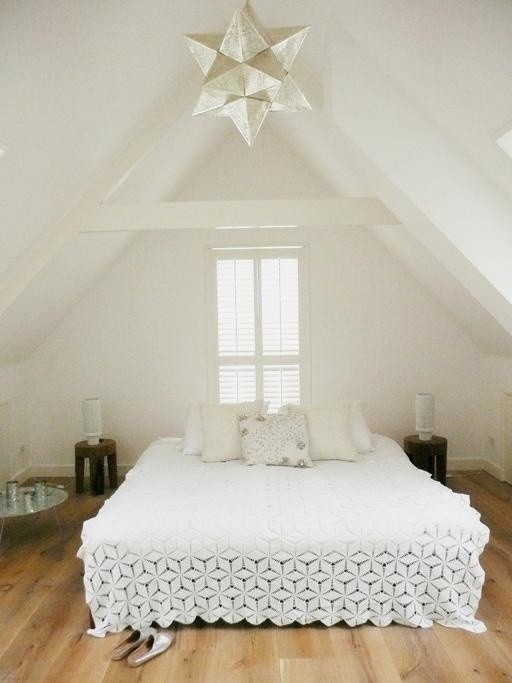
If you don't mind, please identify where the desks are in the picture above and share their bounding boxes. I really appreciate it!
[0,485,70,537]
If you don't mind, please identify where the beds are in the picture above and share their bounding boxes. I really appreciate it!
[90,433,490,630]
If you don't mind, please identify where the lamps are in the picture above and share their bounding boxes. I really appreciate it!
[415,392,436,439]
[183,0,314,147]
[82,398,103,444]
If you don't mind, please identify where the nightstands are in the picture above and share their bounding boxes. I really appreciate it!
[403,435,447,485]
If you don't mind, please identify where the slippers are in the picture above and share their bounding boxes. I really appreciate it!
[110,626,173,668]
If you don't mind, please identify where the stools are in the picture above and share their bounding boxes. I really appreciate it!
[75,438,117,495]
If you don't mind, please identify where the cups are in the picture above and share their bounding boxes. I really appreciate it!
[22,490,38,511]
[33,478,48,498]
[5,480,20,501]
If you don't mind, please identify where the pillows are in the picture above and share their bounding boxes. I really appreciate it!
[182,399,375,467]
